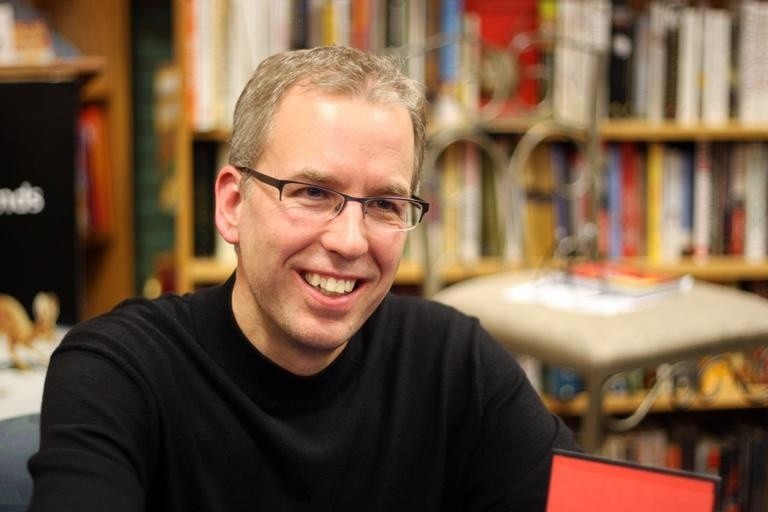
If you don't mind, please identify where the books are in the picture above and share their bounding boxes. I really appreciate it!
[176,1,768,512]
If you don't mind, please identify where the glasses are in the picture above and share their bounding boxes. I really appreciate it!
[236,166,430,232]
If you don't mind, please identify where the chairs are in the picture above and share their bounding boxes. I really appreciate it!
[169,0,768,421]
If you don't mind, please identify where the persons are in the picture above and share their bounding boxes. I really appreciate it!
[30,44,581,509]
